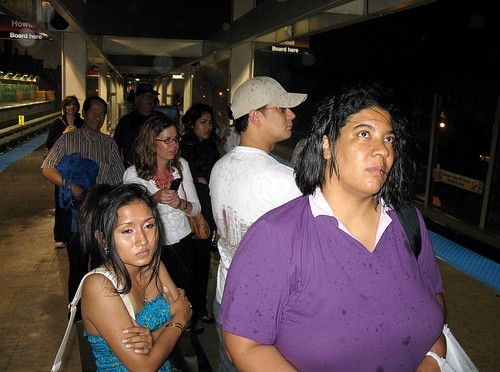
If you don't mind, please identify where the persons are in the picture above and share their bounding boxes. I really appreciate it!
[45,95,85,249]
[206,76,304,372]
[120,114,202,372]
[174,102,220,323]
[119,86,161,103]
[216,80,447,371]
[218,109,242,156]
[41,96,126,324]
[77,180,195,372]
[114,84,175,172]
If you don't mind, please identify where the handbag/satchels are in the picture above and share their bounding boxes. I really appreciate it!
[56,152,99,209]
[426,324,479,372]
[186,213,210,240]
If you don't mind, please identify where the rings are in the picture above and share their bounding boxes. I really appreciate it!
[188,301,192,309]
[178,292,186,297]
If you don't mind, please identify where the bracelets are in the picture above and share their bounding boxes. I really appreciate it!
[166,322,184,335]
[180,200,189,211]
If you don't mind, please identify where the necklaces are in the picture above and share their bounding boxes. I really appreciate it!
[130,276,148,303]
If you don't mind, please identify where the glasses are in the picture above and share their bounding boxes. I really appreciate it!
[156,136,181,144]
[257,107,286,112]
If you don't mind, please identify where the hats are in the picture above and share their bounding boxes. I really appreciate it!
[132,83,160,96]
[230,76,308,120]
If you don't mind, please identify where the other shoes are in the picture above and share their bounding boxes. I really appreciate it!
[55,240,65,248]
[202,310,215,323]
[193,324,204,335]
